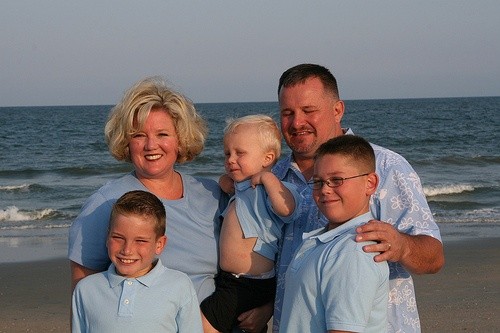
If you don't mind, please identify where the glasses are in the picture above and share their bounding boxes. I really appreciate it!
[307,173,370,190]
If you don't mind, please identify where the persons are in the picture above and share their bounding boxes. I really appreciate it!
[278,134,391,332]
[71,190,205,332]
[67,81,275,333]
[200,113,302,333]
[269,64,445,332]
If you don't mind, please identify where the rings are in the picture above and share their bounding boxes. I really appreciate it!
[241,328,248,333]
[386,242,392,251]
